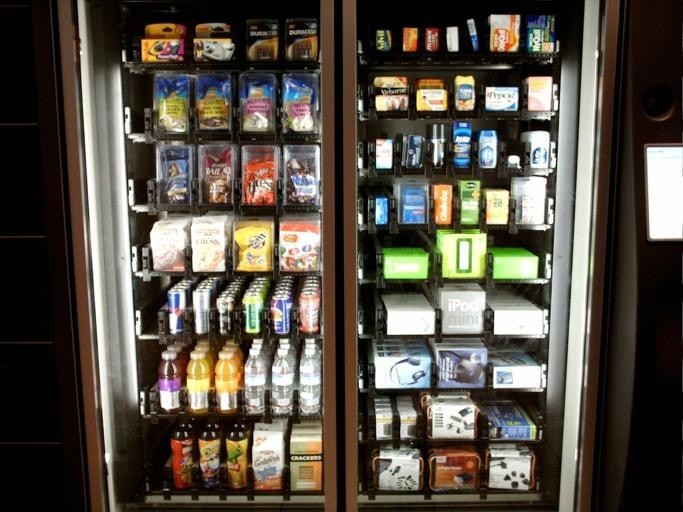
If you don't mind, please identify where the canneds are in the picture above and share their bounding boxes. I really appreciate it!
[166,276,322,335]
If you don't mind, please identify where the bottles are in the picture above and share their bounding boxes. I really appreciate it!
[169,419,250,493]
[156,339,324,416]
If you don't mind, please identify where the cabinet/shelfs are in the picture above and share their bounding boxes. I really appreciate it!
[55,3,340,512]
[340,0,621,510]
